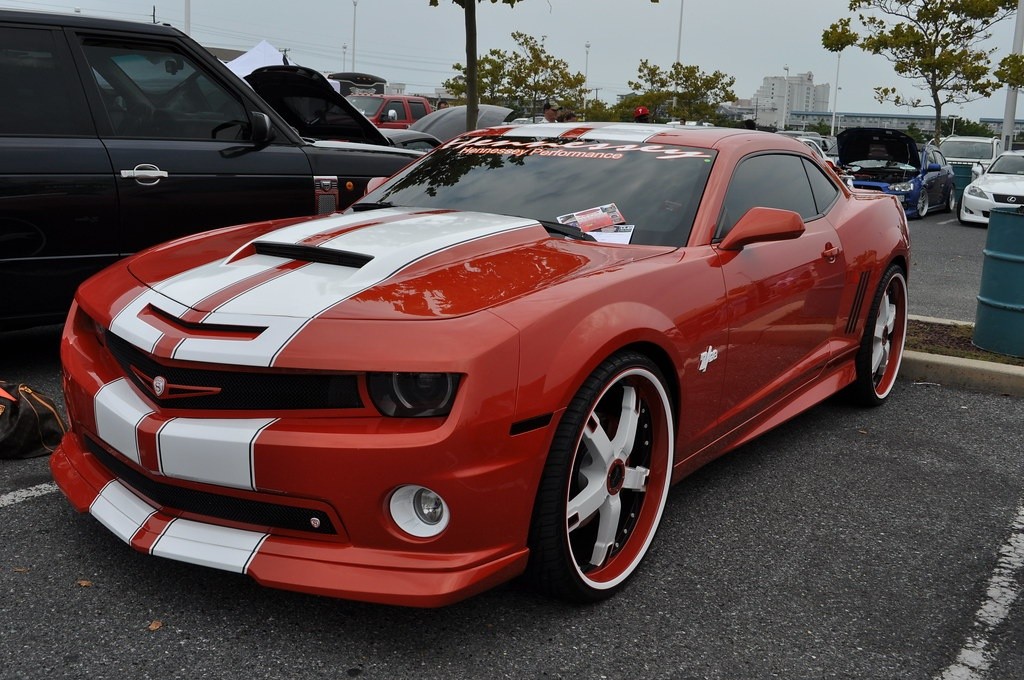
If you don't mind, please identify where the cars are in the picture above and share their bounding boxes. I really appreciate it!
[956,149,1024,226]
[834,127,955,220]
[247,65,515,153]
[777,130,838,172]
[50,120,909,607]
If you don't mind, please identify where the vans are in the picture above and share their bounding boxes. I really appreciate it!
[340,91,434,131]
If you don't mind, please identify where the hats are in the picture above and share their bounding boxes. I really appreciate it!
[635,106,649,117]
[543,101,563,111]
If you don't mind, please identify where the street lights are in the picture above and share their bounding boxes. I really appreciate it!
[585,39,593,105]
[784,65,789,122]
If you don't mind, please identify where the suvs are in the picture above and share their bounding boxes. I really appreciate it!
[1,9,430,348]
[937,134,1001,176]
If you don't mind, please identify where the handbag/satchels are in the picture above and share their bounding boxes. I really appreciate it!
[0,379,68,459]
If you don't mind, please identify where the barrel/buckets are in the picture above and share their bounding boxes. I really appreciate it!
[972,207,1023,358]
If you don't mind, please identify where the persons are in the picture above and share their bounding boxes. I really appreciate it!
[437,101,449,110]
[537,101,563,123]
[563,110,577,122]
[680,119,686,125]
[634,107,649,123]
